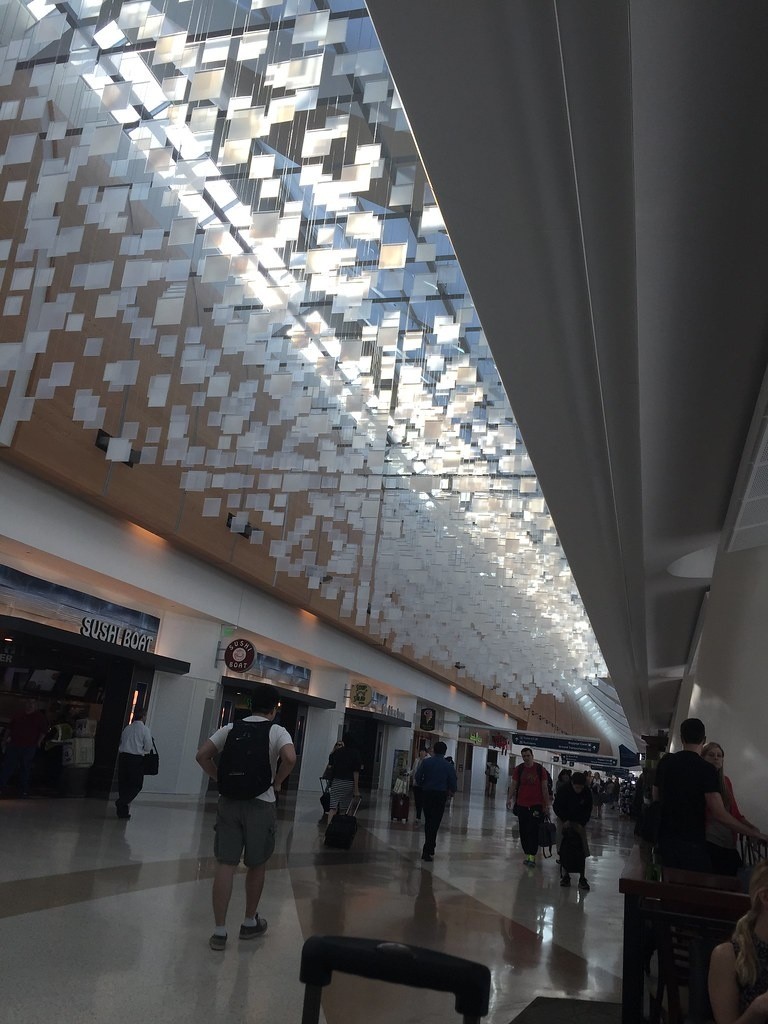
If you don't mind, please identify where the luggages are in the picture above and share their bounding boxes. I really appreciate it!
[319,776,331,814]
[391,773,409,822]
[323,795,362,850]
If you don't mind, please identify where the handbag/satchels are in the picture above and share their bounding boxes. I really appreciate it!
[494,766,500,778]
[142,737,159,775]
[633,800,663,842]
[484,765,491,776]
[539,815,557,846]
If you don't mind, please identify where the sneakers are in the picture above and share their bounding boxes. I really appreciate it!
[560,873,571,887]
[578,878,590,889]
[240,913,268,938]
[522,854,530,864]
[209,932,228,951]
[529,855,536,866]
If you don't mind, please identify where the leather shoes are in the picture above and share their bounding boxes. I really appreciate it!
[422,854,434,862]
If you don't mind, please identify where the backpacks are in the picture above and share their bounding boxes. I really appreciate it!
[217,718,278,799]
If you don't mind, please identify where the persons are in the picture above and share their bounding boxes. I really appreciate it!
[403,747,432,823]
[115,709,153,819]
[488,761,499,788]
[196,685,296,950]
[327,732,361,824]
[701,742,760,875]
[708,858,768,1024]
[556,769,572,790]
[0,697,48,798]
[553,772,593,889]
[445,756,454,765]
[653,718,768,871]
[329,741,344,765]
[632,767,660,880]
[506,748,548,867]
[45,714,72,751]
[584,771,620,819]
[414,741,457,862]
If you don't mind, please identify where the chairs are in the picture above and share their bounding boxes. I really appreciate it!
[690,941,716,1024]
[662,868,745,1024]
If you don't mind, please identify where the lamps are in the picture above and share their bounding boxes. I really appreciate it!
[455,662,465,669]
